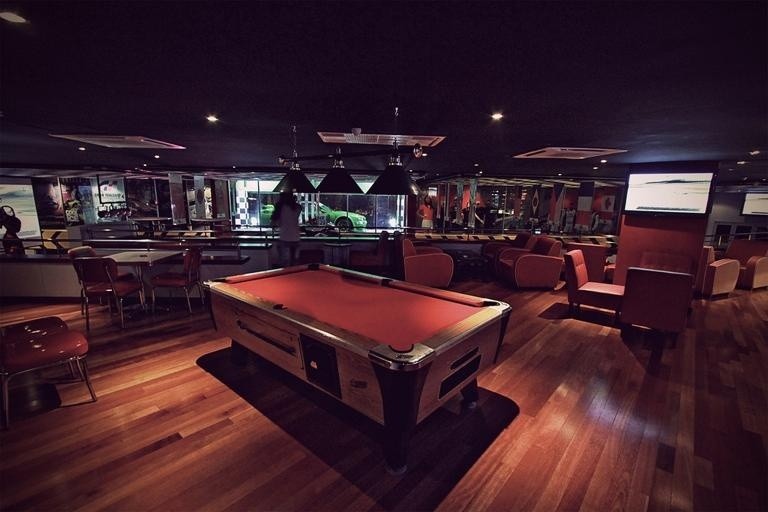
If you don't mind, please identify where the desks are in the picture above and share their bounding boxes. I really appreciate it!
[202,265,511,474]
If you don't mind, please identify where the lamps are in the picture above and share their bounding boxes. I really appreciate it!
[273,126,423,197]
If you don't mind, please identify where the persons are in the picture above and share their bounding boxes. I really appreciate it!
[416,195,437,231]
[588,206,601,234]
[561,202,578,234]
[269,190,305,267]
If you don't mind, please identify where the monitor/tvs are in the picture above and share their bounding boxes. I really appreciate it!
[739,192,768,216]
[622,170,717,218]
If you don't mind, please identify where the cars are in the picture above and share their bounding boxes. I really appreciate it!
[258,198,368,233]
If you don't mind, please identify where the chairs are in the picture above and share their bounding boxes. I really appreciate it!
[149,247,202,314]
[0,316,97,428]
[393,231,454,288]
[73,257,147,332]
[695,245,741,300]
[563,249,625,321]
[480,233,563,289]
[620,267,694,329]
[68,246,131,318]
[350,230,389,272]
[725,239,768,292]
